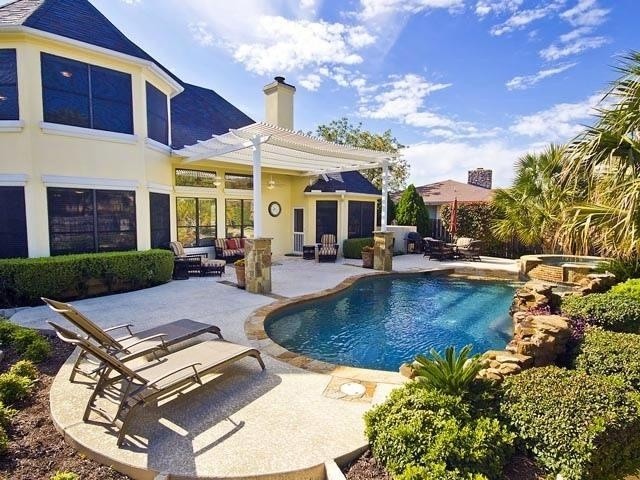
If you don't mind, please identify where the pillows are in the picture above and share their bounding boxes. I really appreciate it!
[226,239,245,249]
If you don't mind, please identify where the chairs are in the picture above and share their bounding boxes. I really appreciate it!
[318,233,339,263]
[169,242,226,277]
[421,235,482,262]
[41,297,265,448]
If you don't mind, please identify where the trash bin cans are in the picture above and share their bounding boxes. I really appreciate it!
[407,232,422,254]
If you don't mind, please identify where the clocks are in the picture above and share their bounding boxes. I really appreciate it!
[268,201,281,217]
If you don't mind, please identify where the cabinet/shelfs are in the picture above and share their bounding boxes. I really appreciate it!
[302,245,315,259]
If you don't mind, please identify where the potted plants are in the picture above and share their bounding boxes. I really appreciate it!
[234,258,245,288]
[361,246,374,269]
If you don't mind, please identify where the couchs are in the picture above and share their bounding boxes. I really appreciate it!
[215,238,255,263]
